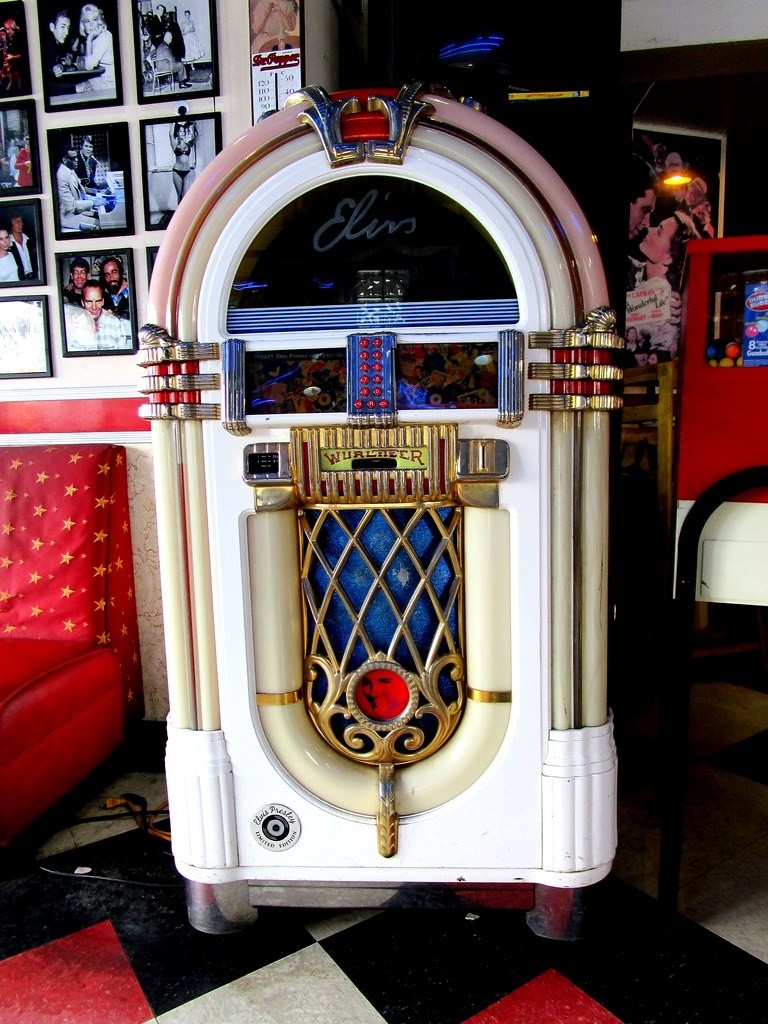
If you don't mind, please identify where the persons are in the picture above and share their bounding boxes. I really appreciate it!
[625,136,714,369]
[0,20,26,98]
[149,4,205,89]
[0,136,32,188]
[169,121,202,205]
[62,257,132,351]
[0,212,39,282]
[56,135,109,231]
[40,4,116,97]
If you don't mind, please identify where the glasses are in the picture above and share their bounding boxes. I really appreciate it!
[65,156,78,161]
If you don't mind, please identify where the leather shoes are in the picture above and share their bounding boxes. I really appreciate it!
[179,83,192,89]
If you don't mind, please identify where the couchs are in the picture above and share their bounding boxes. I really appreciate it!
[0,443,142,846]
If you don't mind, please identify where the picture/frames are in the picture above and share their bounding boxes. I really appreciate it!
[132,0,221,106]
[0,295,55,379]
[47,121,136,241]
[630,118,727,363]
[37,0,125,112]
[140,112,222,231]
[0,199,48,288]
[53,247,140,357]
[0,0,32,101]
[0,98,43,199]
